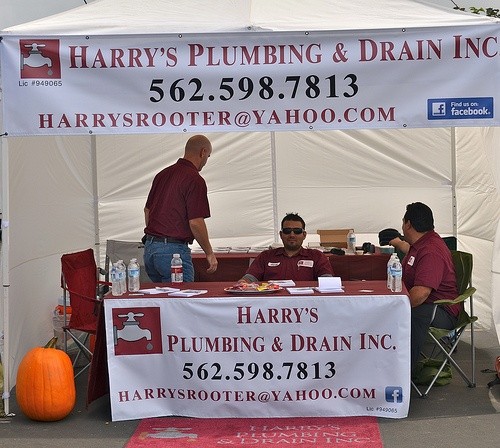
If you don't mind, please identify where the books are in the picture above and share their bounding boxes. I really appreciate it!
[138,286,207,296]
[269,279,344,295]
[190,246,347,253]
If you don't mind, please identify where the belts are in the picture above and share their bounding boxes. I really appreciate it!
[146,234,188,246]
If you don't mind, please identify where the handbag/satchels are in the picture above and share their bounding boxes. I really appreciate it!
[414,360,451,386]
[52,305,91,367]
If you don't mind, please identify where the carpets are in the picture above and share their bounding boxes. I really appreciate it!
[123,417,384,448]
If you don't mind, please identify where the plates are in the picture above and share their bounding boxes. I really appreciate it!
[224,286,283,293]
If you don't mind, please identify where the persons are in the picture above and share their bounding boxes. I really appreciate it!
[144,134,217,282]
[388,202,461,384]
[240,214,332,282]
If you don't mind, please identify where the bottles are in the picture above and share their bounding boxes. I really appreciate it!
[346,230,357,255]
[170,254,183,286]
[110,258,141,297]
[387,252,400,289]
[390,259,403,292]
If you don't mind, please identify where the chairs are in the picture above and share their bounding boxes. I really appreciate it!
[97,239,153,294]
[60,249,108,380]
[378,229,479,399]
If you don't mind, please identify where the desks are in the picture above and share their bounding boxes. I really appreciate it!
[84,282,412,422]
[191,247,391,283]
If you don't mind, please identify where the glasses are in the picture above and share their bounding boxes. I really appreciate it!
[281,227,305,234]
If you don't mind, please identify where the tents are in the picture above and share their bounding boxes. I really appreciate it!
[0,0,500,417]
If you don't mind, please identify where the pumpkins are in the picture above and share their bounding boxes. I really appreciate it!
[16,336,75,421]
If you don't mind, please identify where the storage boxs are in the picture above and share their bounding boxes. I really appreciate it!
[317,228,354,250]
[52,298,90,367]
